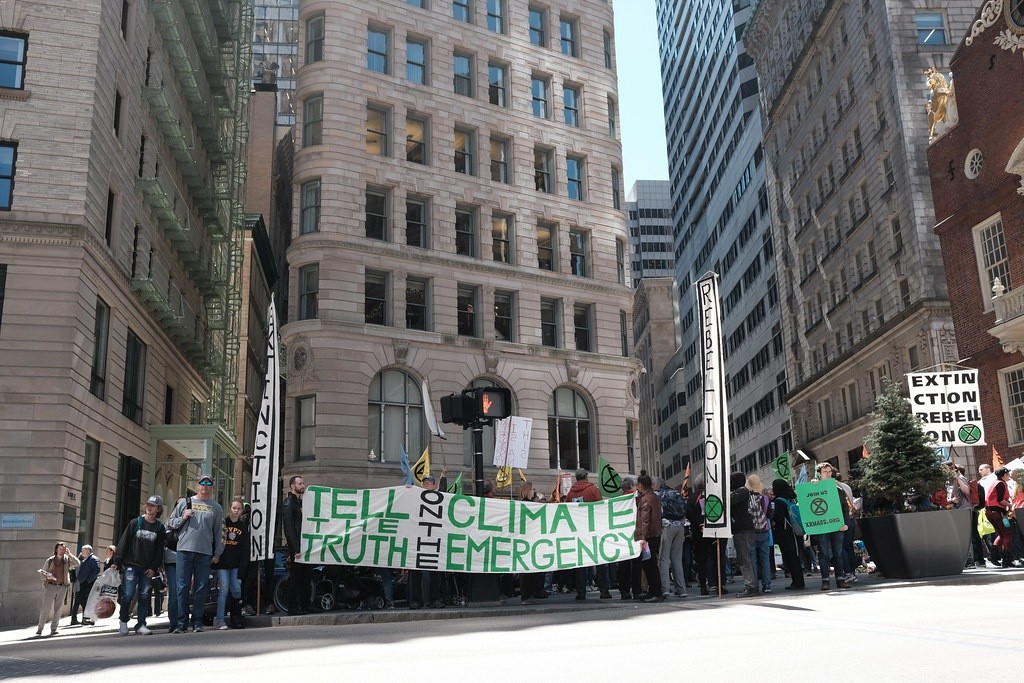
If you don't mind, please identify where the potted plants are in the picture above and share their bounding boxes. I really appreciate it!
[850,376,973,578]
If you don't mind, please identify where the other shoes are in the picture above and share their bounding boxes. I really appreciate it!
[785,585,805,590]
[36,630,41,634]
[135,623,152,635]
[821,580,830,590]
[701,586,709,595]
[644,597,664,603]
[173,624,188,634]
[965,563,975,568]
[51,632,58,634]
[836,579,851,588]
[213,621,228,630]
[241,606,256,616]
[120,620,129,634]
[987,545,1001,566]
[1000,550,1015,568]
[522,596,543,605]
[192,623,204,633]
[762,584,771,593]
[736,588,760,598]
[974,561,986,566]
[600,591,612,599]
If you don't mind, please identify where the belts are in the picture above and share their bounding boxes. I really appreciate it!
[48,581,64,586]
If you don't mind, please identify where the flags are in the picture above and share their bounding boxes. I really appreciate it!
[815,460,818,478]
[496,465,512,488]
[796,463,807,483]
[992,444,1004,471]
[447,470,463,495]
[400,443,430,487]
[771,451,792,482]
[518,469,526,484]
[598,454,624,498]
[862,444,870,458]
[682,462,690,493]
[549,472,560,502]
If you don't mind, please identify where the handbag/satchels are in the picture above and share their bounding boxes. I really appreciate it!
[977,506,996,539]
[83,568,122,619]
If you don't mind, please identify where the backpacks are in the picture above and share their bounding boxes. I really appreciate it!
[654,485,686,521]
[68,567,78,583]
[730,486,768,531]
[775,497,806,537]
[957,478,985,510]
[164,497,192,552]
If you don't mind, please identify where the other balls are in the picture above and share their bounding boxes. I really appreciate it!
[95,597,115,619]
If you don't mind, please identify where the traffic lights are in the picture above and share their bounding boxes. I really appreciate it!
[475,387,511,419]
[440,393,475,424]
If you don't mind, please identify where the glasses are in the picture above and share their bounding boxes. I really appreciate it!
[531,488,535,490]
[199,481,212,486]
[821,471,833,474]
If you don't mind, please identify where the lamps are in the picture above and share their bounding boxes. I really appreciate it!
[791,449,812,468]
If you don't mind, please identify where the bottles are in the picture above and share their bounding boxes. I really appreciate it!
[1002,516,1010,527]
[774,545,783,570]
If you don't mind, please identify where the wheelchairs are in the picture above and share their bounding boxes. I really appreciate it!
[344,571,407,610]
[272,548,334,612]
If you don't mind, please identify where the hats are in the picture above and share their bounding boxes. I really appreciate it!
[145,495,163,505]
[422,476,435,485]
[745,474,763,492]
[995,467,1011,478]
[575,468,589,478]
[199,474,213,483]
[107,545,116,552]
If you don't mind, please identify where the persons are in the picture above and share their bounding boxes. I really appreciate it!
[34,542,81,639]
[70,545,168,625]
[111,495,166,635]
[282,460,1024,616]
[163,496,275,633]
[168,473,227,634]
